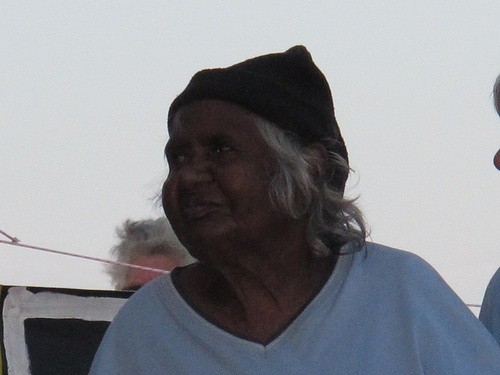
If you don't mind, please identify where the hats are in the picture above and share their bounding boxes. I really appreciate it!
[168,44,348,156]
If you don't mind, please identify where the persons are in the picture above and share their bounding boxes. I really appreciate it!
[103,217,200,292]
[86,46,500,375]
